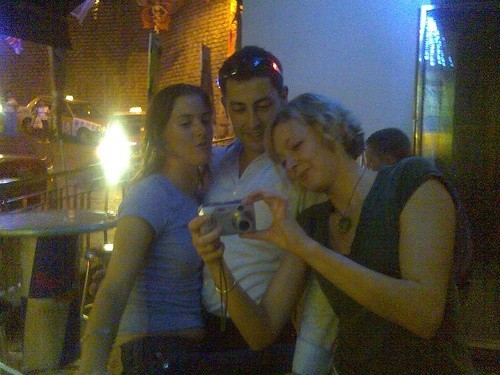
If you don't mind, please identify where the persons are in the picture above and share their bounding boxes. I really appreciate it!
[76,84,213,375]
[196,45,333,375]
[366,127,413,171]
[189,92,475,375]
[3,90,18,136]
[36,98,51,144]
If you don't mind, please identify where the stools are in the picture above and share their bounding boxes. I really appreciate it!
[78,245,114,330]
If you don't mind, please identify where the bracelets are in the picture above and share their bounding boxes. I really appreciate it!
[215,279,236,294]
[79,328,117,342]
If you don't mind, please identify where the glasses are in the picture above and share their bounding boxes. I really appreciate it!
[219,56,283,101]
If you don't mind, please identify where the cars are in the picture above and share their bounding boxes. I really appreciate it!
[17,93,107,147]
[96,107,148,165]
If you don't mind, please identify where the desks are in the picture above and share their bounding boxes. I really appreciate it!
[0,210,121,375]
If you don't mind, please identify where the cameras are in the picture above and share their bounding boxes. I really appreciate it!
[198,200,258,236]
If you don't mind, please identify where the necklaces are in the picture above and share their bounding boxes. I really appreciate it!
[335,169,366,234]
